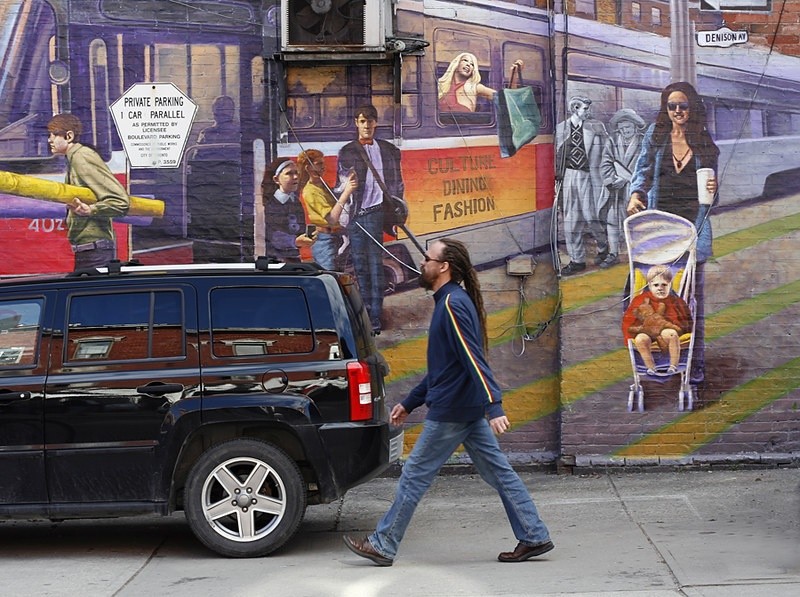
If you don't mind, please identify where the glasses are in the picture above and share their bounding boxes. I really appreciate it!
[424,254,449,262]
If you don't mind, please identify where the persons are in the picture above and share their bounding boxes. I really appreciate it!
[342,239,554,567]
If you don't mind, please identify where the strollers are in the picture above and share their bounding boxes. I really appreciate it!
[623,203,703,413]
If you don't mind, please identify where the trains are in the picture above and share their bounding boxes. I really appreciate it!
[0,0,794,294]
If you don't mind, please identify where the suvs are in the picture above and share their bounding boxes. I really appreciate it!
[0,254,407,559]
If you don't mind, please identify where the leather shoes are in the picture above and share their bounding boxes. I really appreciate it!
[343,536,393,566]
[498,539,554,561]
